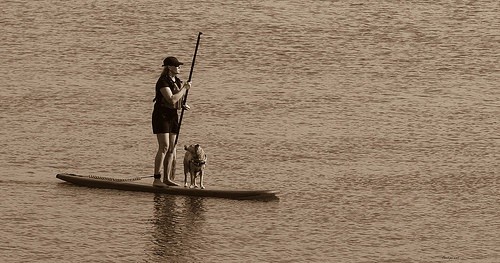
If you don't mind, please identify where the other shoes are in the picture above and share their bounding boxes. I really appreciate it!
[164,180,181,186]
[153,181,168,186]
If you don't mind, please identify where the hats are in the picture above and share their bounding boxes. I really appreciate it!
[163,57,184,67]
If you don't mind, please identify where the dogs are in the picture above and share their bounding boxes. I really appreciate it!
[183,143,208,189]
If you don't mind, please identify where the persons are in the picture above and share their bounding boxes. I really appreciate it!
[151,56,192,187]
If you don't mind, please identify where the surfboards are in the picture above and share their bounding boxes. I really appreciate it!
[56,172,280,201]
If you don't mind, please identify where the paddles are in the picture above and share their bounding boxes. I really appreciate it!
[170,31,203,179]
